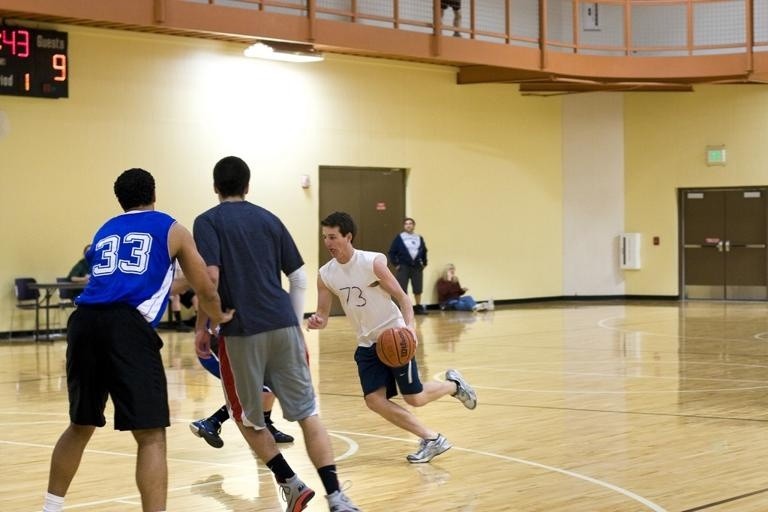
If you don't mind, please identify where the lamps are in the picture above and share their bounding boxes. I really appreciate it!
[242,38,323,65]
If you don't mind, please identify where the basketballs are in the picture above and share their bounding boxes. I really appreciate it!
[376,328,416,368]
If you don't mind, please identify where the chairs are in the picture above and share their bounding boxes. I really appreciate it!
[7,276,46,344]
[54,276,78,340]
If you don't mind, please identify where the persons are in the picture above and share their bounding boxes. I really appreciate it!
[189,311,294,448]
[37,167,237,512]
[193,155,367,512]
[306,211,477,464]
[435,264,494,314]
[387,218,428,313]
[169,256,199,332]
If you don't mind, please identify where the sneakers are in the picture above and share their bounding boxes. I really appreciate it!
[278,474,315,512]
[323,488,363,512]
[406,433,454,464]
[471,303,487,313]
[265,422,295,444]
[175,322,191,334]
[415,304,429,314]
[189,418,224,450]
[445,368,478,410]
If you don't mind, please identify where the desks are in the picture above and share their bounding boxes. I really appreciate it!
[23,280,181,345]
